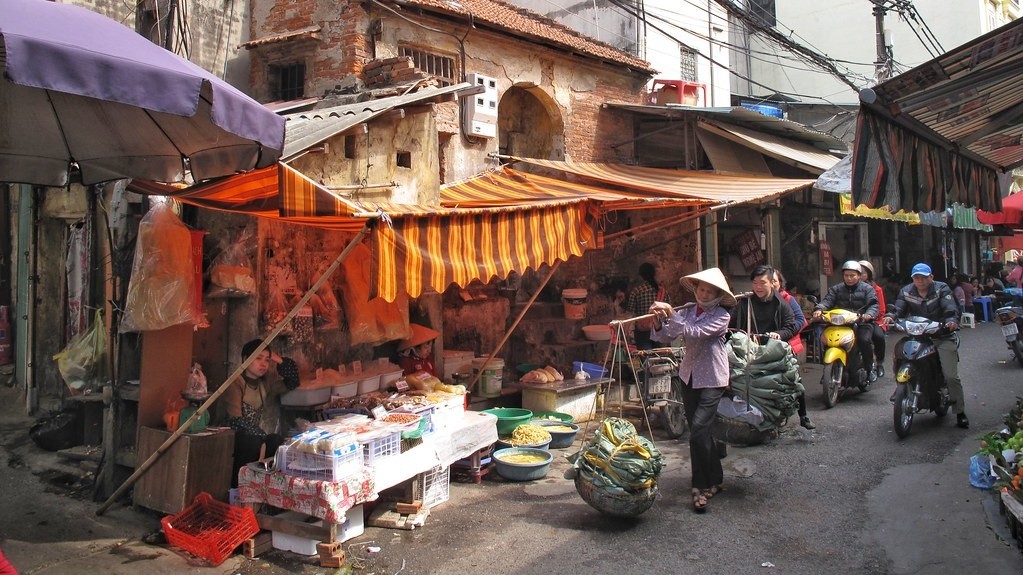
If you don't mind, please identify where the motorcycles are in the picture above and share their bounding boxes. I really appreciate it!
[638,345,686,439]
[878,303,961,440]
[806,294,879,408]
[993,286,1023,366]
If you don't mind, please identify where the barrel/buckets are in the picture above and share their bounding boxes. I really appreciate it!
[573,360,609,378]
[561,289,588,320]
[472,358,505,397]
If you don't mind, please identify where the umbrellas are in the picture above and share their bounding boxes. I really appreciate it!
[977,189,1023,230]
[0,0,287,190]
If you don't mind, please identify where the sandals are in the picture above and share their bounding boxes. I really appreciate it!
[703,484,723,500]
[692,489,709,510]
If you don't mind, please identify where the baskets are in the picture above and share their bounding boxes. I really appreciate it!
[161,491,261,565]
[278,446,364,483]
[361,430,402,466]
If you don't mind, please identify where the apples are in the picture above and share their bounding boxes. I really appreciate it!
[996,430,1023,454]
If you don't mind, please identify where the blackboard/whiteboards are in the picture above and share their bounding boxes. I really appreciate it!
[733,229,769,275]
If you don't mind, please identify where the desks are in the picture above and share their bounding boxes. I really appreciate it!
[237,411,497,535]
[800,324,829,363]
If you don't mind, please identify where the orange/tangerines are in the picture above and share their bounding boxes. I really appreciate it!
[1012,468,1023,490]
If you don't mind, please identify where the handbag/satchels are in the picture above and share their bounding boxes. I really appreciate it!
[637,285,665,331]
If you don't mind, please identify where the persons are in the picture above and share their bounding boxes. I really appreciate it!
[393,322,441,381]
[649,268,738,508]
[813,260,880,383]
[771,270,816,430]
[224,338,300,488]
[944,256,1023,326]
[883,263,969,428]
[726,265,797,347]
[626,261,670,360]
[787,281,805,308]
[882,269,902,304]
[858,260,886,378]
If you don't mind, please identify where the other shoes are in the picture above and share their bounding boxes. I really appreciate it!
[799,416,816,429]
[876,364,885,377]
[957,412,969,425]
[868,370,877,382]
[890,386,898,401]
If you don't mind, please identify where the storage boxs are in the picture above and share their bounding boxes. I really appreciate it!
[796,339,806,363]
[228,489,264,515]
[160,493,260,565]
[271,384,464,556]
[523,387,598,425]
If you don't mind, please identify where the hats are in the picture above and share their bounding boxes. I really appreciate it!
[397,324,438,352]
[679,267,738,306]
[910,264,932,277]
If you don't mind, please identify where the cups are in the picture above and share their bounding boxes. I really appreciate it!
[973,288,978,298]
[166,412,179,432]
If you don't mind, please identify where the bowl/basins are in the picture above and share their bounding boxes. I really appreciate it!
[531,421,580,449]
[481,408,533,435]
[492,447,553,480]
[533,412,575,425]
[516,363,547,379]
[981,296,987,299]
[496,432,552,454]
[581,325,612,340]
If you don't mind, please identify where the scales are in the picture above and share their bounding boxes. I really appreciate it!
[613,335,631,364]
[181,391,211,433]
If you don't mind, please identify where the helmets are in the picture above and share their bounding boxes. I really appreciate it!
[841,260,862,273]
[858,259,873,274]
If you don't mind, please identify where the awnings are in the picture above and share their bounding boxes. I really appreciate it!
[851,16,1023,214]
[700,121,920,226]
[953,202,993,233]
[95,154,605,518]
[488,146,819,268]
[919,204,953,228]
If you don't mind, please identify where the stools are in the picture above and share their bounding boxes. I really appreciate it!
[961,314,975,328]
[649,80,707,105]
[972,298,994,321]
